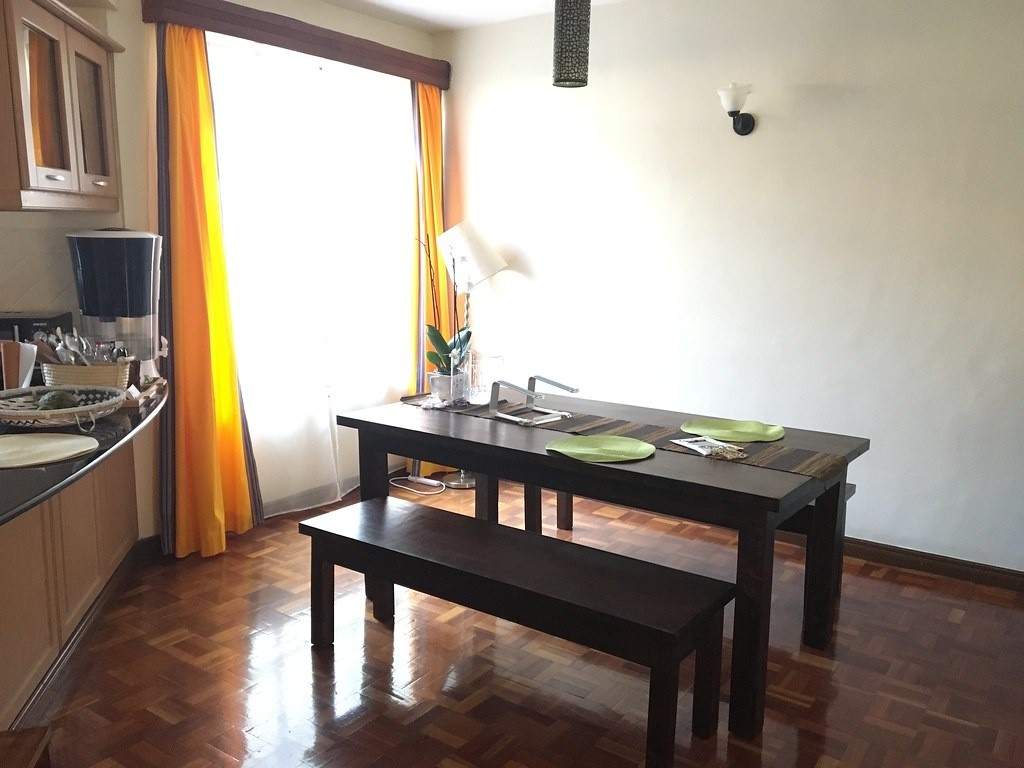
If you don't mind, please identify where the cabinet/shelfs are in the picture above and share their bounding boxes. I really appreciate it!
[0,0,126,213]
[0,434,147,732]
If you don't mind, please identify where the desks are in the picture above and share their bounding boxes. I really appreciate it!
[337,382,872,746]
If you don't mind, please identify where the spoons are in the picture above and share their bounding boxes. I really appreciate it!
[33,329,118,366]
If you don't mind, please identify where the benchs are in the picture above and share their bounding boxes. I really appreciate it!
[300,495,740,767]
[464,473,858,625]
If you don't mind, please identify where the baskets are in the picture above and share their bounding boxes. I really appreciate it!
[42,362,130,391]
[0,384,127,433]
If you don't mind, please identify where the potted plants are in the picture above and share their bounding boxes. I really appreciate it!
[419,233,472,400]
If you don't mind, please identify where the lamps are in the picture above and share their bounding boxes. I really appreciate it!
[717,83,755,136]
[438,220,508,489]
[552,0,590,89]
